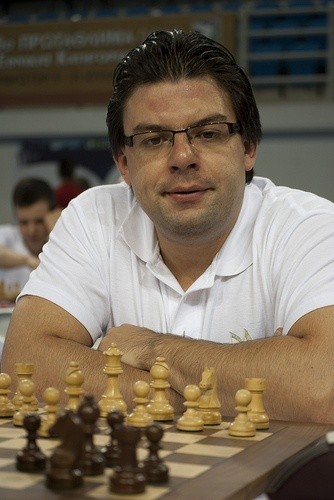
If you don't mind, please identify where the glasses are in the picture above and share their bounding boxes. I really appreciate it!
[121,121,240,151]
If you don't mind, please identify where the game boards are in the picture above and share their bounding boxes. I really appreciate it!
[0,402,334,500]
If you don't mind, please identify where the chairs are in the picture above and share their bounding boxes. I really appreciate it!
[0,0,334,98]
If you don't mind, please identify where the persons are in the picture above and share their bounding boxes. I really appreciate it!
[0,174,62,296]
[0,29,334,425]
[52,159,83,208]
